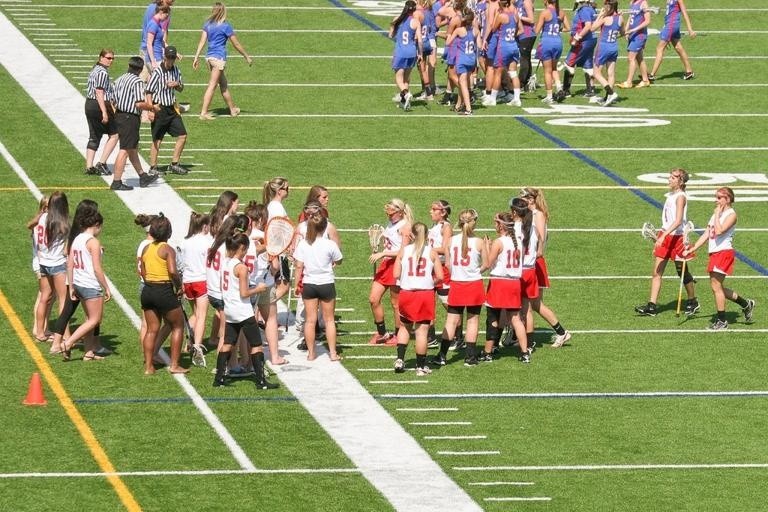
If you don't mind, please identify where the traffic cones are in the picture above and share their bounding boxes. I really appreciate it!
[20,367,48,408]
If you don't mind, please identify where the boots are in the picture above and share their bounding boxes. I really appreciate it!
[250,352,280,389]
[213,351,232,386]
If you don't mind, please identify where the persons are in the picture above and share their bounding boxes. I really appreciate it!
[35,191,85,344]
[25,196,60,340]
[639,0,696,80]
[633,167,700,319]
[84,47,119,176]
[110,57,161,190]
[192,2,253,121]
[144,46,189,177]
[56,207,112,363]
[138,0,175,85]
[681,186,756,332]
[388,0,650,116]
[367,187,571,377]
[133,177,344,390]
[48,198,115,356]
[145,3,183,77]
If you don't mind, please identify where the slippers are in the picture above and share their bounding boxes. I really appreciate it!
[31,329,105,366]
[259,317,345,367]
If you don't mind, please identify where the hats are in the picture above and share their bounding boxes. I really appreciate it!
[165,46,177,58]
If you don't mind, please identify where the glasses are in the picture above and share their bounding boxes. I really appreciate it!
[386,210,397,219]
[520,189,534,198]
[280,187,289,191]
[494,214,514,226]
[460,210,479,225]
[431,201,451,211]
[104,56,115,61]
[671,169,688,183]
[718,195,727,201]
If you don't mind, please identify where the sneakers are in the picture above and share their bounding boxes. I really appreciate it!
[364,330,573,377]
[635,302,657,316]
[110,181,133,190]
[231,107,240,117]
[685,297,700,316]
[707,319,728,330]
[96,162,112,175]
[200,114,217,120]
[84,167,97,175]
[684,73,694,80]
[140,174,157,187]
[740,299,756,322]
[169,162,187,175]
[639,75,655,83]
[179,104,190,112]
[391,77,650,117]
[149,169,163,177]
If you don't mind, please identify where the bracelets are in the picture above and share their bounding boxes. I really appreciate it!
[176,82,181,89]
[150,104,155,113]
